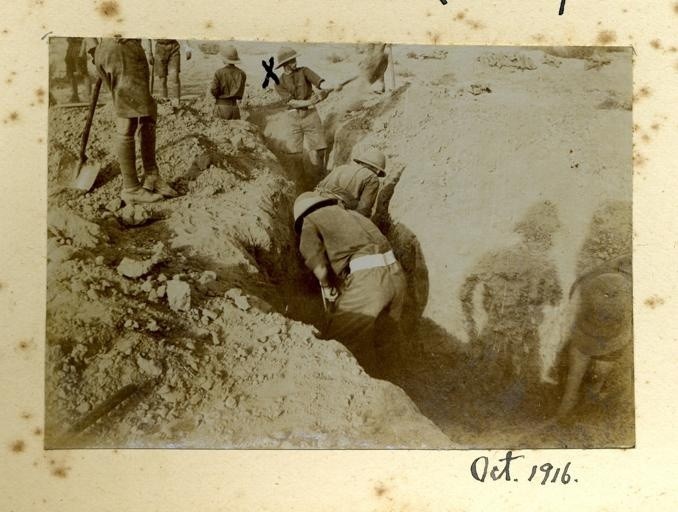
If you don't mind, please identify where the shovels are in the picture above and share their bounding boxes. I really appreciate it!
[69,77,102,192]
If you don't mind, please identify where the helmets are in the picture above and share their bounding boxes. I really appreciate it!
[569,273,632,354]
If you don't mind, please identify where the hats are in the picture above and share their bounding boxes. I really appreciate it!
[352,147,388,178]
[274,45,302,71]
[220,44,241,65]
[292,190,339,232]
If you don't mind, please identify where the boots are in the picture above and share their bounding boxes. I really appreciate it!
[139,128,180,197]
[117,140,164,206]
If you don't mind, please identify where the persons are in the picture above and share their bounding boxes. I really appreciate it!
[85,38,178,202]
[212,45,247,121]
[62,38,93,104]
[146,40,181,99]
[308,145,388,218]
[295,191,404,373]
[274,45,345,179]
